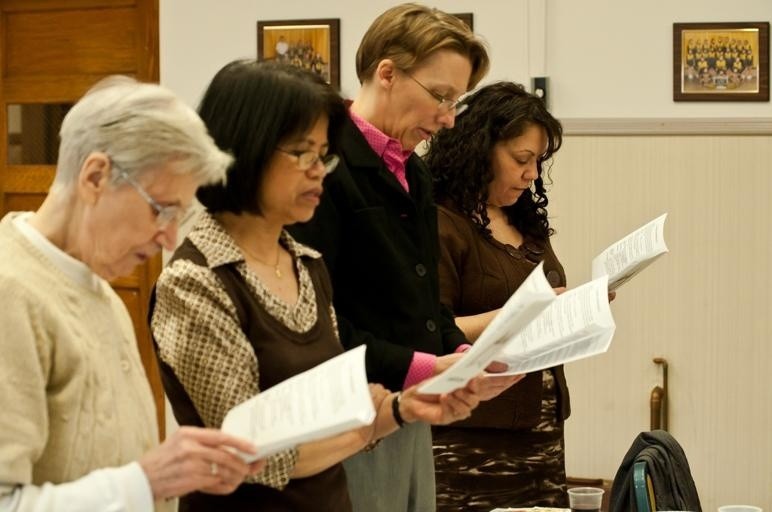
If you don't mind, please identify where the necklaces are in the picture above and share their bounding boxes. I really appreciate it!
[242,244,283,279]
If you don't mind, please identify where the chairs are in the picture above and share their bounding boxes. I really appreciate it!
[625,441,687,511]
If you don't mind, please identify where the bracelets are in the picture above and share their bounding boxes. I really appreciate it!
[391,389,409,429]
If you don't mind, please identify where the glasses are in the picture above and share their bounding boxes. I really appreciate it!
[274,148,341,174]
[110,159,196,234]
[395,66,470,116]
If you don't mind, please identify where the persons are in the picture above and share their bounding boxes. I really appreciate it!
[1,74,270,509]
[685,34,756,91]
[282,4,492,512]
[275,35,328,78]
[418,79,621,510]
[146,56,528,511]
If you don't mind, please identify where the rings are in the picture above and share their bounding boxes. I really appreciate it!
[211,461,218,476]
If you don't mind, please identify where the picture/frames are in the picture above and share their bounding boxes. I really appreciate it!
[673,21,770,102]
[256,17,341,92]
